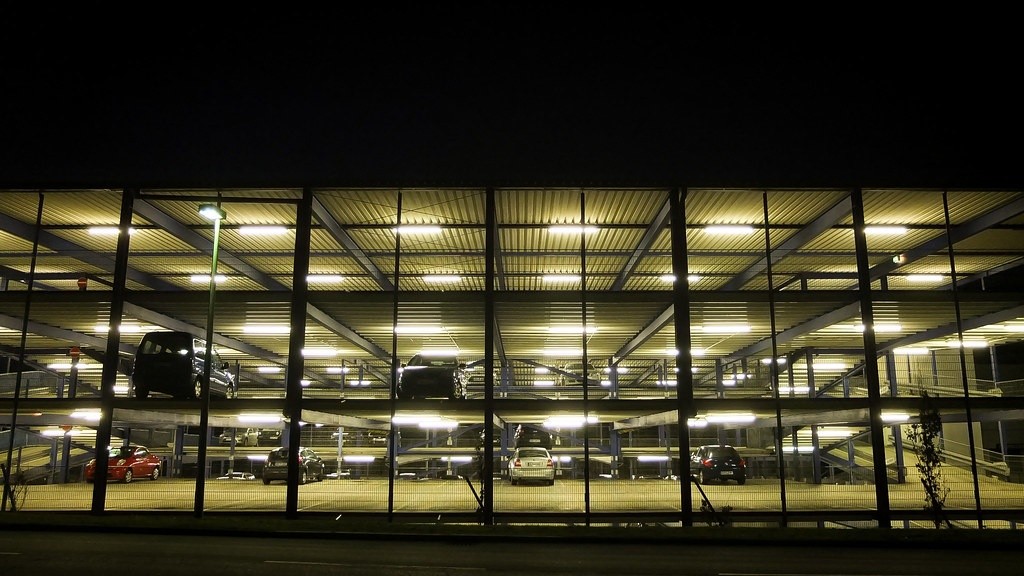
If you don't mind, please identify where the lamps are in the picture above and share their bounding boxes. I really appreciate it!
[198,203,227,222]
[893,253,900,264]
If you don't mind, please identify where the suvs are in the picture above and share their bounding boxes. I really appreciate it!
[265,445,325,487]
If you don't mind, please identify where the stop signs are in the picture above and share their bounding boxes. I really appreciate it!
[77,277,88,288]
[69,347,81,360]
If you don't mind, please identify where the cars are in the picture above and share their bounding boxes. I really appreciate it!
[514,423,553,449]
[554,361,601,387]
[396,351,470,400]
[86,443,162,487]
[256,427,282,446]
[218,426,260,447]
[331,426,389,446]
[217,471,255,481]
[509,448,557,484]
[690,444,749,486]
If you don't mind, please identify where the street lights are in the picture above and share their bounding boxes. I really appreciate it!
[194,204,227,529]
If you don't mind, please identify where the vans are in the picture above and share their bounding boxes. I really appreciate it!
[132,331,238,407]
